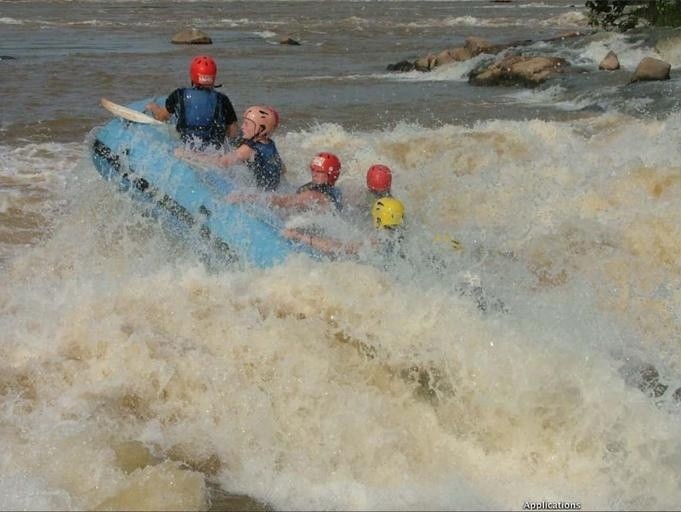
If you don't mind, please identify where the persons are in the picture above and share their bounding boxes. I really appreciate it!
[145,55,239,152]
[224,152,343,216]
[283,164,406,254]
[174,105,287,193]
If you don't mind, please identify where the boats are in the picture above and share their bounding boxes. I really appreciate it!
[84,93,527,334]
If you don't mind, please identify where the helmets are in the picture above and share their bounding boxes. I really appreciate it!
[432,233,463,255]
[366,164,392,193]
[370,196,405,232]
[243,106,279,143]
[191,56,216,86]
[309,153,340,186]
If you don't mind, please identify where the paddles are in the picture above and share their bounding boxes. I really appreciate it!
[99,97,178,127]
